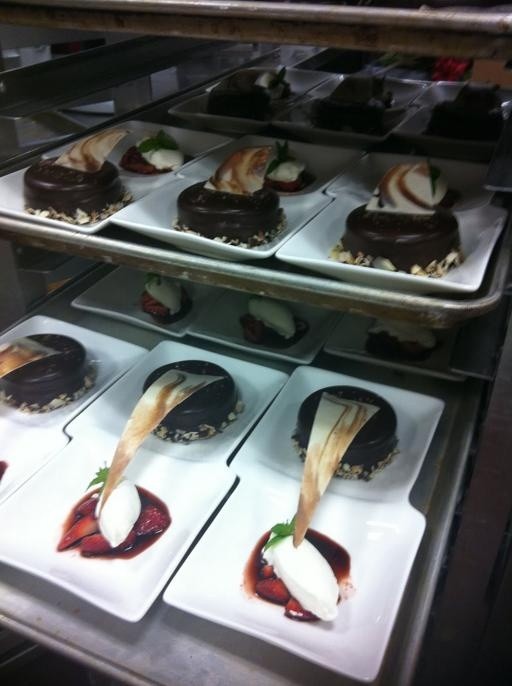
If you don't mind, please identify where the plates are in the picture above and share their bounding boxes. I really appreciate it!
[0,309,448,681]
[68,264,495,394]
[1,38,511,297]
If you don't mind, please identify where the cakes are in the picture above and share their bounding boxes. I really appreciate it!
[172,145,288,250]
[1,333,96,415]
[205,68,290,123]
[93,360,243,513]
[22,125,132,222]
[418,80,504,140]
[290,385,397,547]
[327,162,464,279]
[309,74,392,134]
